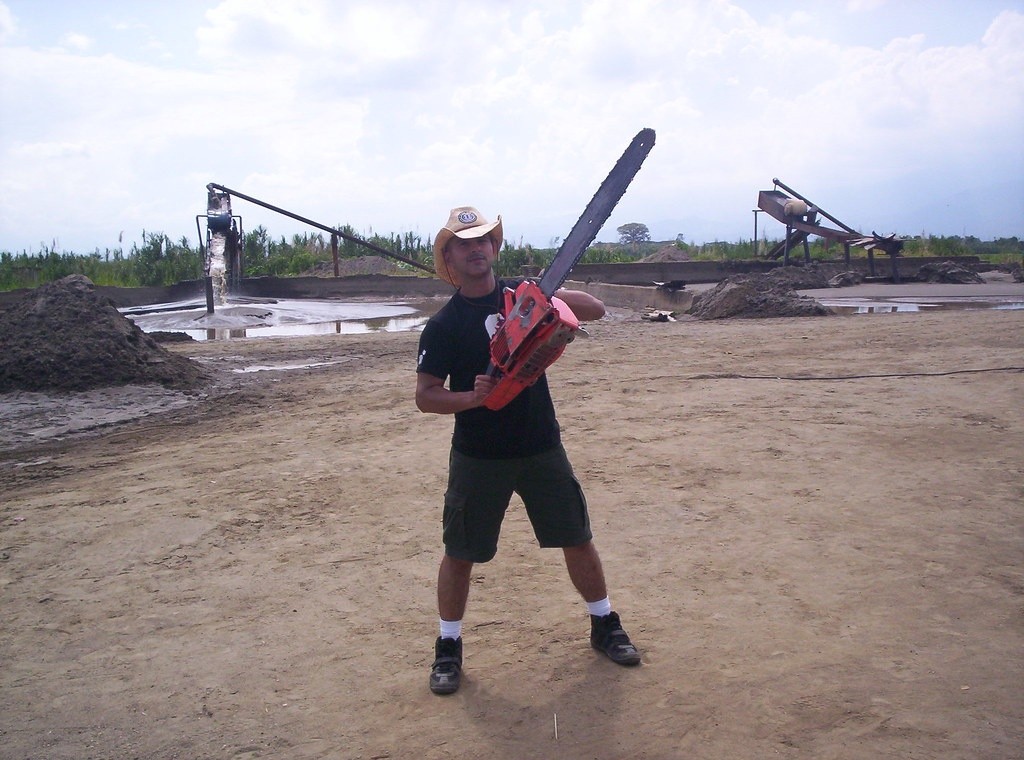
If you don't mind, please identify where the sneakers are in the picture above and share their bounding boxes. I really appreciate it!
[429,635,463,694]
[590,610,641,665]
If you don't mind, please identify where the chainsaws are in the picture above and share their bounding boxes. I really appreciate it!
[480,126,657,412]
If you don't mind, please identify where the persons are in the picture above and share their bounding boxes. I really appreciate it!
[415,207,640,694]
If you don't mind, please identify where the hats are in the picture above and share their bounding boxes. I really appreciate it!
[433,205,504,289]
